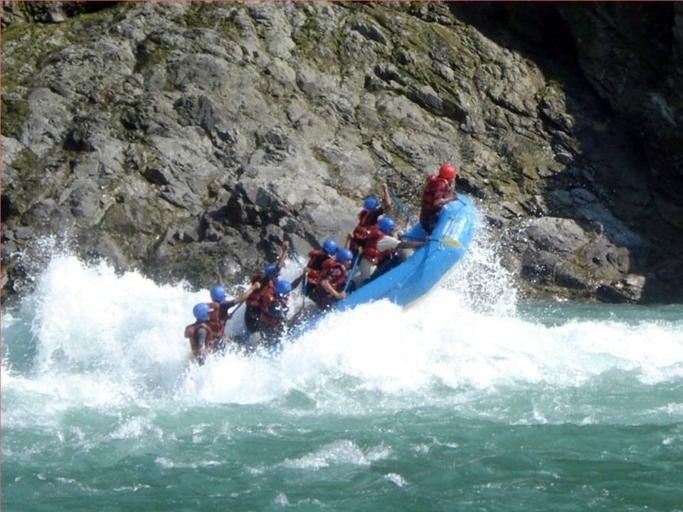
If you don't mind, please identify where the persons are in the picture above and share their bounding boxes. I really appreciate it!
[305,183,433,309]
[419,163,457,234]
[185,240,310,366]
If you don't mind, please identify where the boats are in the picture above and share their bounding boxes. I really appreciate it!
[189,193,479,361]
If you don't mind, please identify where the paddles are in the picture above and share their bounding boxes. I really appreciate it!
[398,236,460,247]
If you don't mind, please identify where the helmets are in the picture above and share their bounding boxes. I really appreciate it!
[210,285,226,303]
[378,217,397,235]
[438,165,456,179]
[364,199,378,210]
[322,239,353,264]
[266,266,275,276]
[273,280,292,295]
[193,303,211,321]
